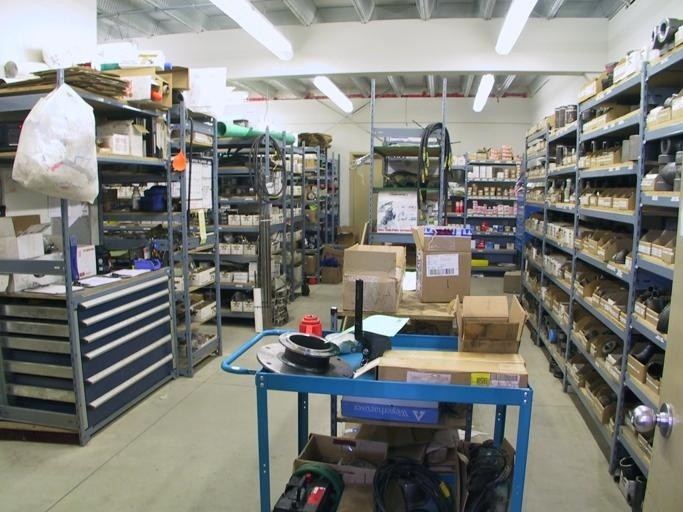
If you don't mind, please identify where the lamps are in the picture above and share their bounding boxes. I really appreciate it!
[495,0,537,56]
[207,0,295,59]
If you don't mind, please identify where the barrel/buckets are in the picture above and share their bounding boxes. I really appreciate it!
[451,168,465,183]
[143,189,166,210]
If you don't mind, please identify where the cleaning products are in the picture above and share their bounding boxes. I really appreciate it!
[131,183,141,211]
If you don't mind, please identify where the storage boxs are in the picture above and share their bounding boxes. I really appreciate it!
[375,347,530,391]
[409,224,477,305]
[449,291,529,354]
[344,241,409,316]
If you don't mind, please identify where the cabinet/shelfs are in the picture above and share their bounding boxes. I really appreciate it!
[1,55,345,450]
[515,54,681,512]
[359,78,521,274]
